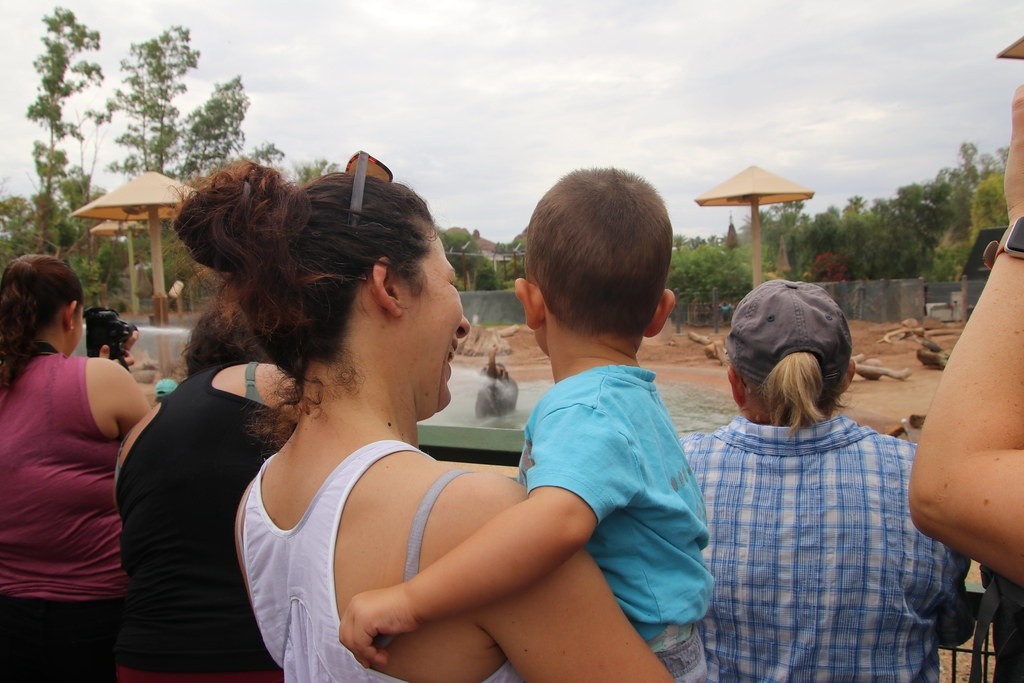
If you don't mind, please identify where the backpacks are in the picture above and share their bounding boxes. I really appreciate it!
[968,564,1024,683]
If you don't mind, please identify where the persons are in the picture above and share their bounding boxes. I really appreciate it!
[176,161,676,683]
[675,280,975,683]
[908,85,1024,682]
[0,254,301,683]
[339,167,716,683]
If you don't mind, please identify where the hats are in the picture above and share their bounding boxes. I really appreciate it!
[725,279,853,392]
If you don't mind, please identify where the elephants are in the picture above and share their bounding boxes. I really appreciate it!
[475,343,518,420]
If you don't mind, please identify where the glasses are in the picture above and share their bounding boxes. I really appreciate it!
[344,151,393,227]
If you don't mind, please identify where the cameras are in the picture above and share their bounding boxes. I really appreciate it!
[81,307,138,361]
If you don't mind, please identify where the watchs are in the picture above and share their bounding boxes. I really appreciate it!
[983,215,1024,269]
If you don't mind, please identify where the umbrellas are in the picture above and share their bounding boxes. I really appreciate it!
[695,166,815,297]
[69,167,197,374]
